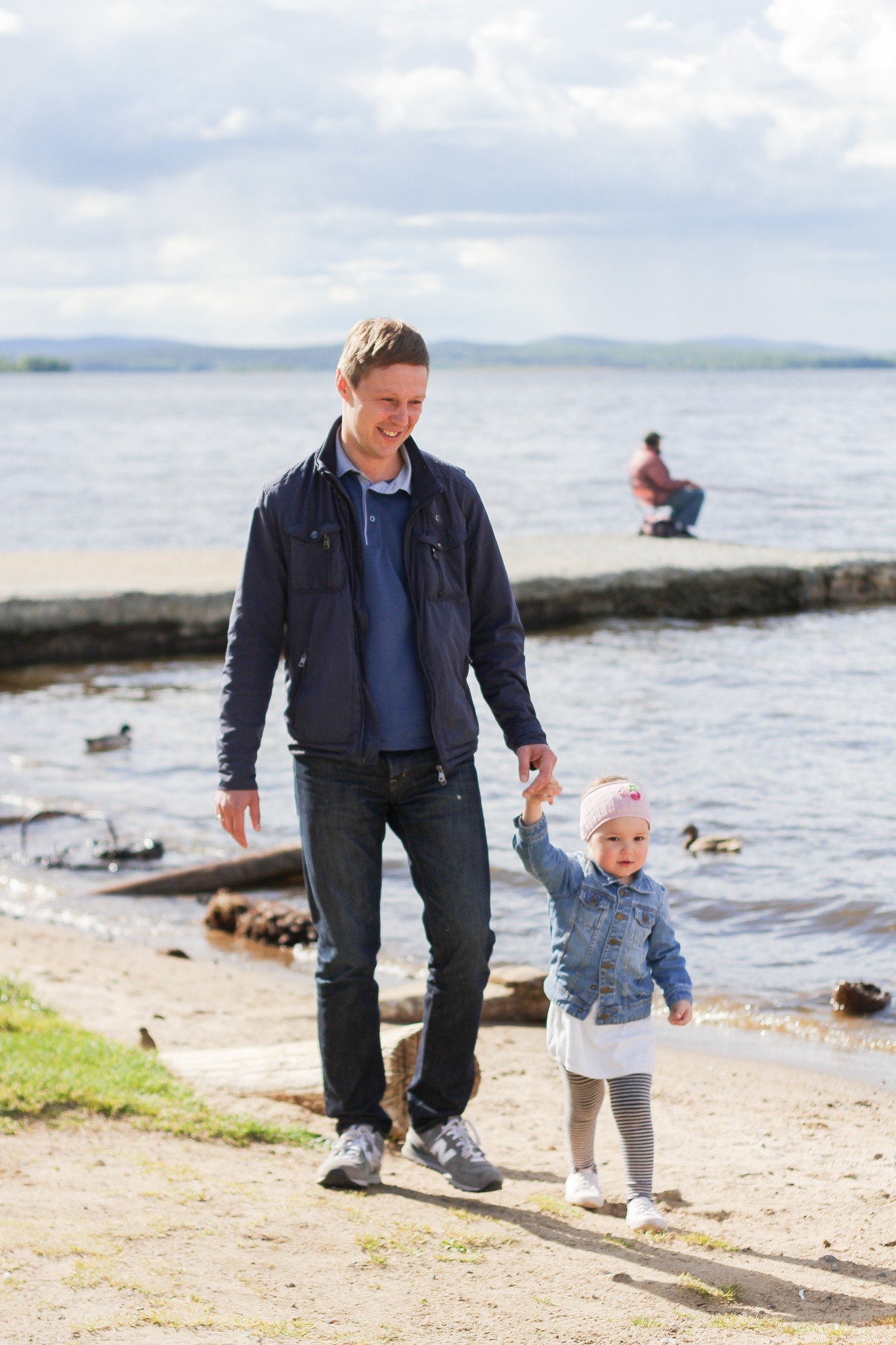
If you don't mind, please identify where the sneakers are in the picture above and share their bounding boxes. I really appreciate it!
[402,1111,503,1194]
[566,1165,605,1207]
[318,1123,385,1189]
[625,1197,666,1229]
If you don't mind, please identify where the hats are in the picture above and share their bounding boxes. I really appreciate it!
[581,774,652,843]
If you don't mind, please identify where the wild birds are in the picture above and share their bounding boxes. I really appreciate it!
[86,725,130,751]
[137,1028,156,1053]
[677,826,741,855]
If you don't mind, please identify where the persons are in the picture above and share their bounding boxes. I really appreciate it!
[630,433,704,539]
[514,776,694,1233]
[213,319,557,1190]
